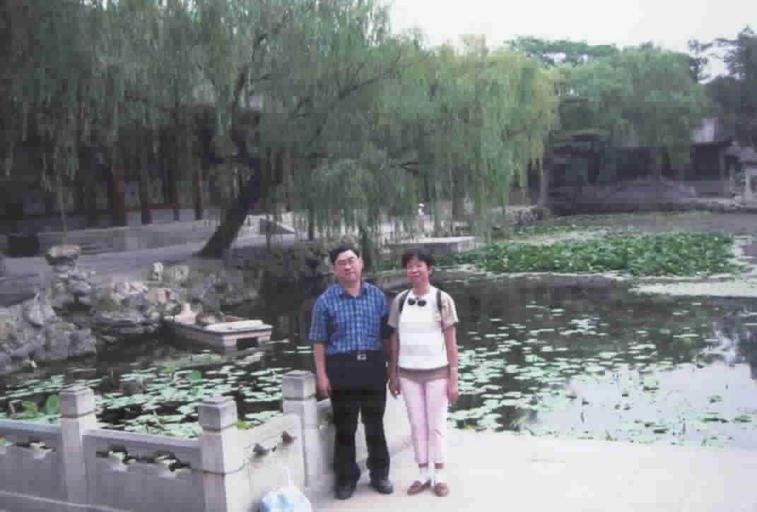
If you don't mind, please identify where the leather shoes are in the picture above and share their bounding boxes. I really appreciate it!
[434,480,448,497]
[371,477,392,493]
[337,486,351,499]
[407,477,431,494]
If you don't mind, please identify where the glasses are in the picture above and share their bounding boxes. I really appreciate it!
[408,298,426,306]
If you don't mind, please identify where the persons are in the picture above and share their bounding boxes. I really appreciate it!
[385,247,459,496]
[308,244,399,500]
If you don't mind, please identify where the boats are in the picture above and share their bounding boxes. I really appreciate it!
[161,297,274,348]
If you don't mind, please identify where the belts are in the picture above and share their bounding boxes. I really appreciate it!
[327,353,384,360]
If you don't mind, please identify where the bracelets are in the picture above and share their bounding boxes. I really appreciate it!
[449,366,457,376]
